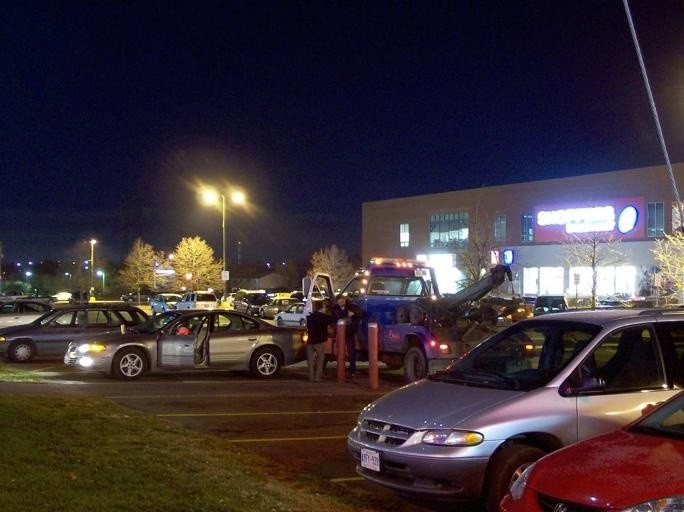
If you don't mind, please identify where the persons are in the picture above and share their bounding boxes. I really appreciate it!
[306,299,337,380]
[331,292,361,375]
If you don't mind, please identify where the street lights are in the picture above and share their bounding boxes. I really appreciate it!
[82,237,96,296]
[193,181,248,306]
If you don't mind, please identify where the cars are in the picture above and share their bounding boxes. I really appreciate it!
[174,291,219,312]
[0,304,150,363]
[63,309,309,381]
[0,299,79,330]
[150,293,182,316]
[522,295,569,317]
[51,292,72,301]
[220,289,303,319]
[345,304,684,511]
[120,290,152,304]
[275,302,305,329]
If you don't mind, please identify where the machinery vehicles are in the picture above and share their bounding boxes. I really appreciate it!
[301,255,512,385]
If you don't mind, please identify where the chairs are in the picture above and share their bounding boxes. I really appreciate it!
[560,339,599,376]
[192,320,236,350]
